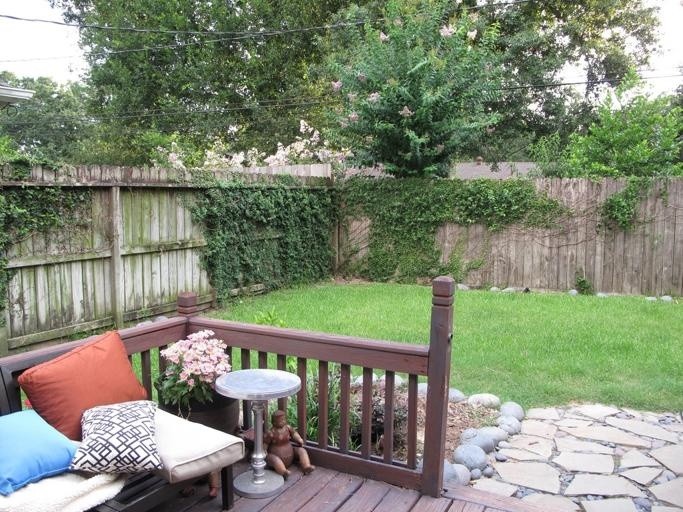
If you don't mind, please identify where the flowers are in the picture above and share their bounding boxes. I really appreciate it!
[154,328,232,420]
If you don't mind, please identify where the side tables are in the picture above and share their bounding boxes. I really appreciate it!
[214,368,302,500]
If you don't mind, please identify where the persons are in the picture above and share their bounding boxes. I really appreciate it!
[263,409,316,481]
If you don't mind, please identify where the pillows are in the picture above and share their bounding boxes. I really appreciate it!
[0,328,166,499]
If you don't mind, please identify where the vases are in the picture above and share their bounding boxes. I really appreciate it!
[164,399,240,434]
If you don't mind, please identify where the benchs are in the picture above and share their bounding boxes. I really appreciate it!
[0,461,235,512]
